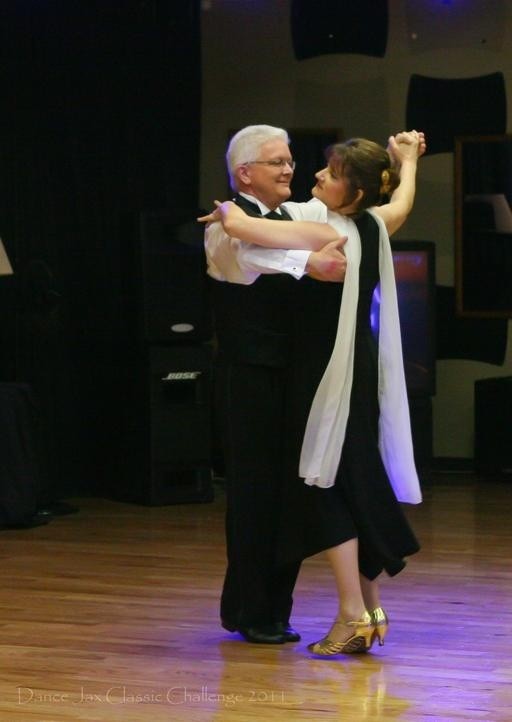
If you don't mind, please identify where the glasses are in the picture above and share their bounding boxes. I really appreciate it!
[238,158,297,172]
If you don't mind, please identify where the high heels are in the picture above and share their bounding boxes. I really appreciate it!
[307,606,390,656]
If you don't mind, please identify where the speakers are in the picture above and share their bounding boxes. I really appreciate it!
[104,344,214,503]
[121,208,213,344]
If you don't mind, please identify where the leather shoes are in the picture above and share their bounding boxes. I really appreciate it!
[2,501,52,528]
[221,615,301,644]
